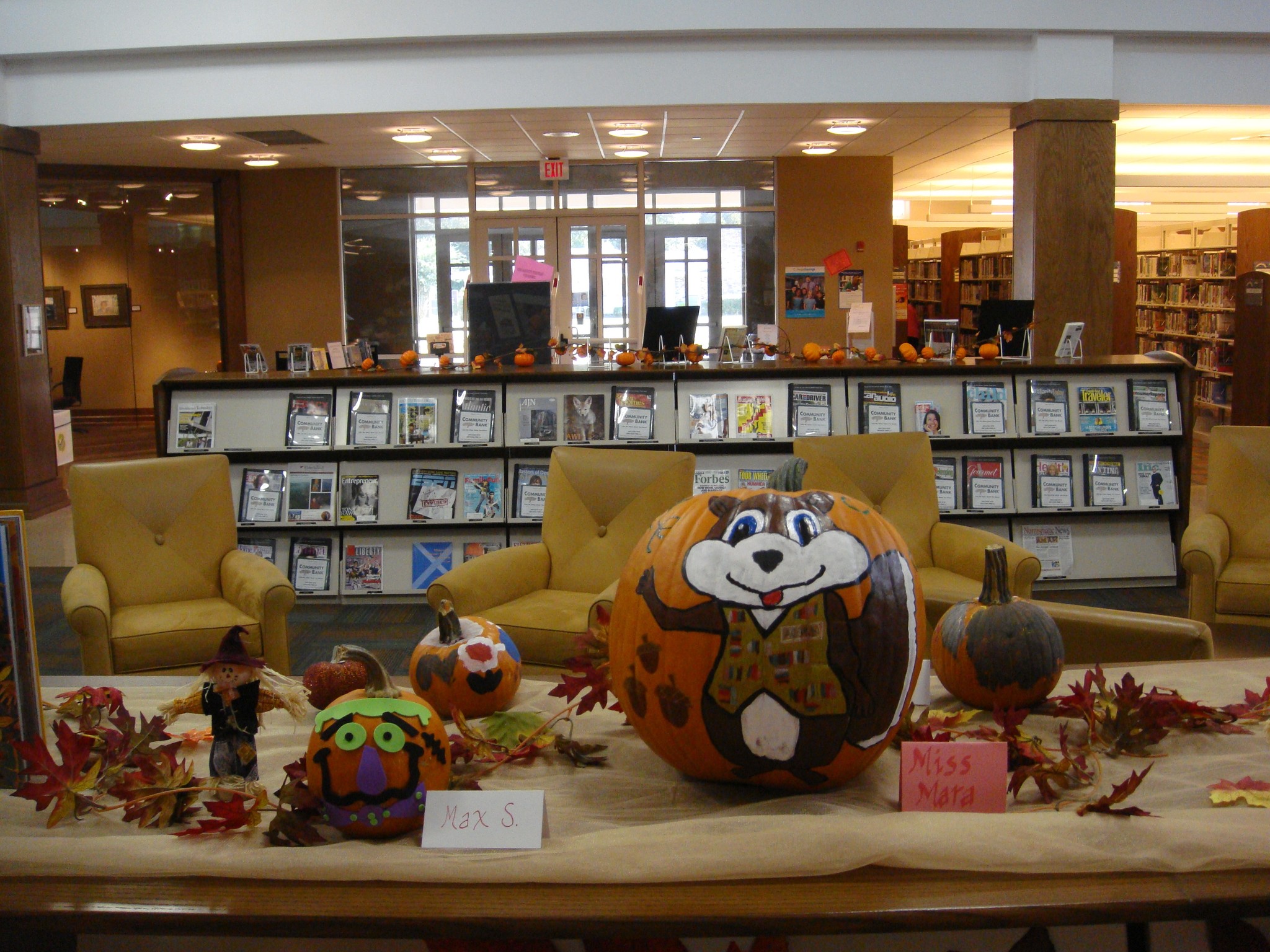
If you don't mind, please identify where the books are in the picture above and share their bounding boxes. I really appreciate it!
[1135,460,1176,507]
[901,251,1017,358]
[1082,451,1127,508]
[173,377,1072,583]
[1135,250,1235,408]
[1126,378,1170,431]
[1076,384,1118,434]
[0,509,47,792]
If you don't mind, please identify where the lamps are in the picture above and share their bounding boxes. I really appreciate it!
[802,144,836,155]
[181,137,221,151]
[244,155,279,166]
[608,124,648,138]
[427,149,462,161]
[392,128,432,142]
[614,146,649,158]
[826,121,866,135]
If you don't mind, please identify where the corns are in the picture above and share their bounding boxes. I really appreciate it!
[173,686,285,715]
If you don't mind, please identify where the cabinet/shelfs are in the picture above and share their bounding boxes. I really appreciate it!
[908,230,959,349]
[1138,207,1270,425]
[959,209,1136,352]
[153,352,1196,606]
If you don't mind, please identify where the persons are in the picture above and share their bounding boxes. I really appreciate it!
[156,623,308,786]
[1150,465,1164,505]
[841,275,861,292]
[1046,463,1059,477]
[787,275,824,310]
[350,475,380,519]
[923,409,940,435]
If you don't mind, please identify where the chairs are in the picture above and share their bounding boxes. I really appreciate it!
[50,357,88,434]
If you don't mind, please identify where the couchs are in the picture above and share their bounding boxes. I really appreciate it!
[426,446,696,686]
[1180,426,1270,627]
[1013,596,1214,666]
[795,433,1042,666]
[61,453,296,674]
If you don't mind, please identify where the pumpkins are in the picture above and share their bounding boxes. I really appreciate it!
[555,344,567,356]
[303,646,369,709]
[686,343,704,363]
[764,344,776,357]
[439,356,449,368]
[929,544,1064,706]
[514,352,534,368]
[615,351,635,366]
[474,355,485,367]
[408,600,522,718]
[307,644,453,837]
[800,342,999,362]
[361,358,375,369]
[610,461,924,790]
[399,350,419,368]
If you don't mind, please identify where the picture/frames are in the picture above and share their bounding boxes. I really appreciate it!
[43,286,66,328]
[21,303,44,357]
[80,284,131,326]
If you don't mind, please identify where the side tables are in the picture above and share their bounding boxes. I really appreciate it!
[892,225,908,357]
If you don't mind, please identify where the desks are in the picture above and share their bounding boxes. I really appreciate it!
[0,660,1268,952]
